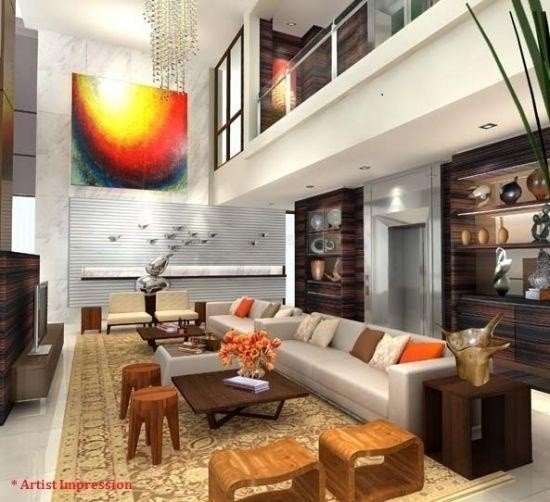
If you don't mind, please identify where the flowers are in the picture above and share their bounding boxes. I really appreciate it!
[218,329,282,378]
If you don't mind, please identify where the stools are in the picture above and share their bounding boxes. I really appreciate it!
[319,418,425,502]
[127,386,180,465]
[208,434,326,502]
[119,363,161,419]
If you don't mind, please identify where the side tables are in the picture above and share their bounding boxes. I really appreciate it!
[423,374,533,480]
[171,365,309,430]
[136,325,205,351]
[151,342,242,387]
[195,301,206,327]
[81,306,101,334]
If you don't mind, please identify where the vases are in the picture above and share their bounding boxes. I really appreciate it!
[311,260,325,282]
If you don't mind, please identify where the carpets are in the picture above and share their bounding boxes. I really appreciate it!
[50,329,515,502]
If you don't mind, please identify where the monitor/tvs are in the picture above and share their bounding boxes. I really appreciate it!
[26,280,53,356]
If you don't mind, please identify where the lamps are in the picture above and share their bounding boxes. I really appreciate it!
[142,0,200,103]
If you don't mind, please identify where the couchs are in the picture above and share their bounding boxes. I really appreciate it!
[259,312,495,440]
[204,299,306,346]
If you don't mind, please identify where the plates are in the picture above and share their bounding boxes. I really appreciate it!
[326,210,342,227]
[310,215,323,230]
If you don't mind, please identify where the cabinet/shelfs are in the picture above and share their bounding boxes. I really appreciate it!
[294,187,364,321]
[441,142,550,370]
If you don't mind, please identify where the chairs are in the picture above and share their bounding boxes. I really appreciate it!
[107,293,152,335]
[155,290,200,326]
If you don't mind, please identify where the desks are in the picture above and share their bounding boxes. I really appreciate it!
[10,323,63,420]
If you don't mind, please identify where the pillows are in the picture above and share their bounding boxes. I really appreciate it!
[228,295,444,375]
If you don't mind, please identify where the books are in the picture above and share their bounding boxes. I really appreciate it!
[222,375,269,391]
[232,386,270,394]
